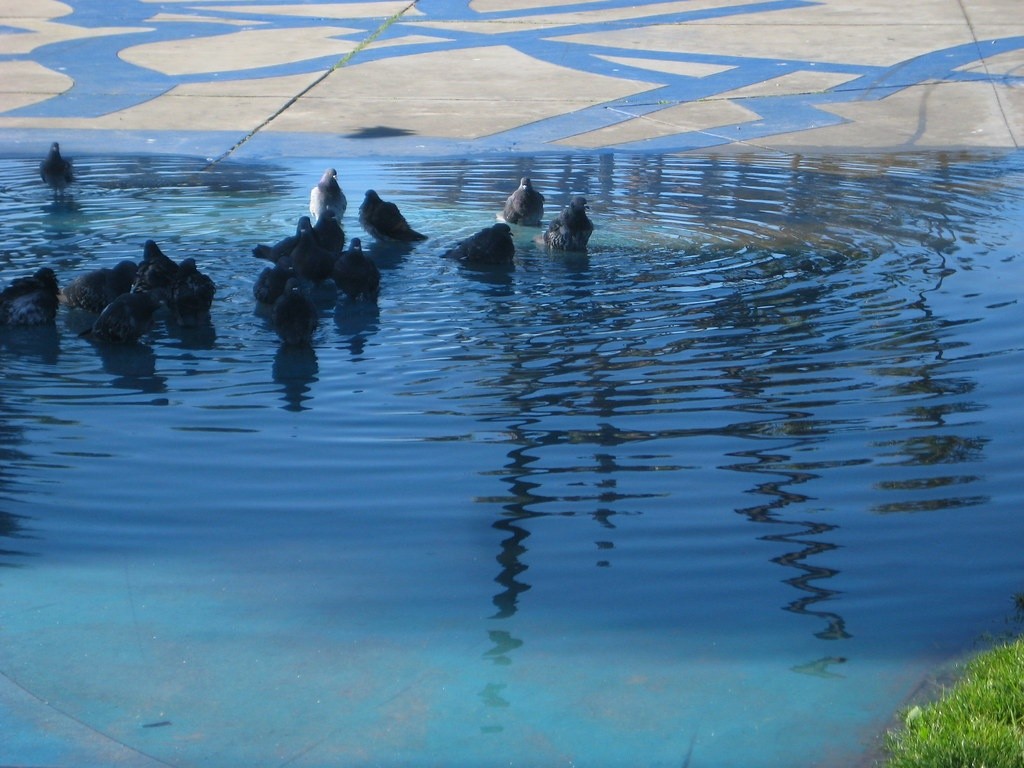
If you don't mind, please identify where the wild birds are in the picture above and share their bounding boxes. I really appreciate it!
[439,223,515,264]
[40,142,76,196]
[57,239,216,348]
[543,197,594,251]
[358,189,428,242]
[250,211,381,350]
[0,267,62,328]
[497,176,546,225]
[309,168,347,225]
